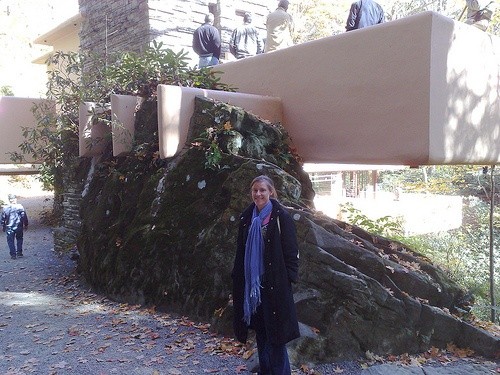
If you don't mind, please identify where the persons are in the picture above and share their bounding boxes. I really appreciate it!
[193,13,221,68]
[345,0,384,32]
[0,194,28,259]
[463,0,496,31]
[264,0,294,53]
[231,175,301,375]
[229,13,264,59]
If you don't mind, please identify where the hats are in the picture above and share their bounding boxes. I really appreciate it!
[8,195,16,199]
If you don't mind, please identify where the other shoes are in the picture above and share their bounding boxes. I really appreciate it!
[11,257,16,259]
[18,256,23,258]
